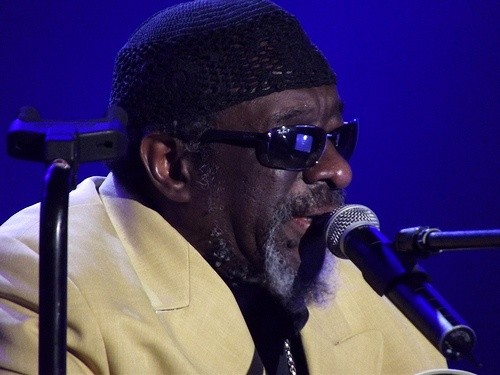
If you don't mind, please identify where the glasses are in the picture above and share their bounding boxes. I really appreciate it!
[161,119,359,171]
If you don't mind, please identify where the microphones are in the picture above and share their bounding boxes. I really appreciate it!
[322,205,479,362]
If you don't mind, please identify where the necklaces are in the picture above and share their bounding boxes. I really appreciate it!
[284,339,296,375]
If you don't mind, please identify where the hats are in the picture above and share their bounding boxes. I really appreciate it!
[110,0,337,124]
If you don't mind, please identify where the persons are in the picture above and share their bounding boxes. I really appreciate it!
[0,0,449,375]
